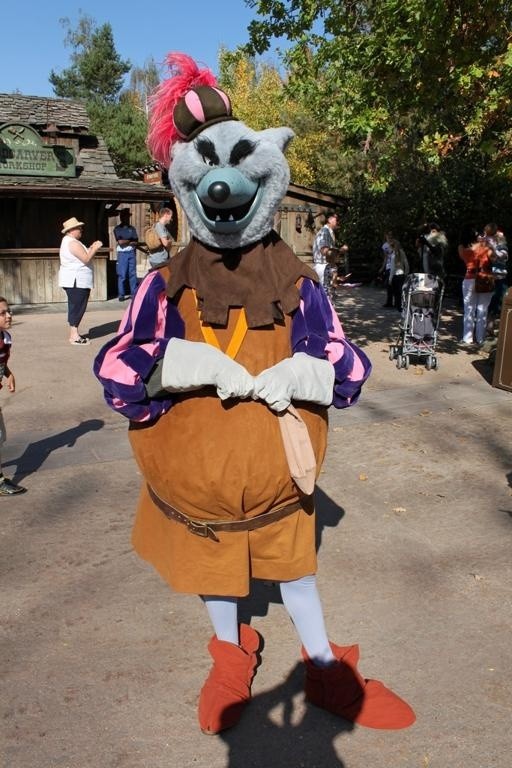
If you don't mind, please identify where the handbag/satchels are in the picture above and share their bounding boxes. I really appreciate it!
[145,228,162,250]
[475,276,495,293]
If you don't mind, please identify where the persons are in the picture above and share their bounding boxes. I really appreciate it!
[312,211,510,347]
[148,207,174,268]
[0,296,26,496]
[58,217,104,346]
[112,208,139,302]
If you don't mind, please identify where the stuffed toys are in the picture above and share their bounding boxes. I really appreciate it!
[92,53,418,736]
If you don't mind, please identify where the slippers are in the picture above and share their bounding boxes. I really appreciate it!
[69,335,90,344]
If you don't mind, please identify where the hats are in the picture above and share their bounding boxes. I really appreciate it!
[61,216,84,233]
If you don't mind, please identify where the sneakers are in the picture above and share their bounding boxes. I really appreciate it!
[0,477,25,495]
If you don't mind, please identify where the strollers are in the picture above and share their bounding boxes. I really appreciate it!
[389,270,445,371]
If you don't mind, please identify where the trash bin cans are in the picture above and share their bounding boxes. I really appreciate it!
[492,286,512,393]
